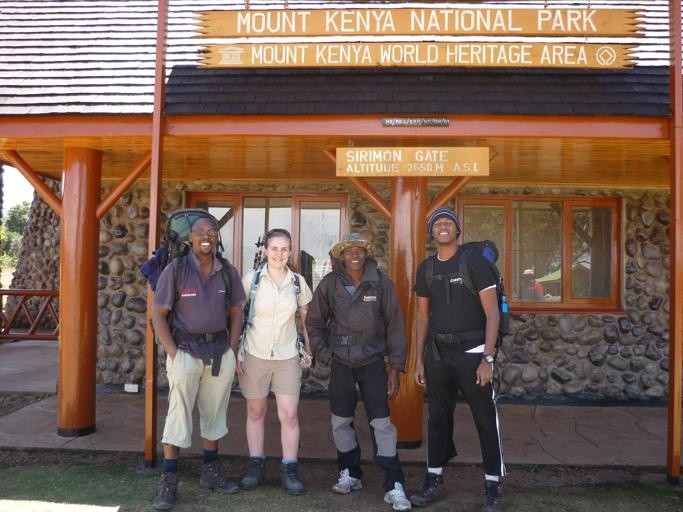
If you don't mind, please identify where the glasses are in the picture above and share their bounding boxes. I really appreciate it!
[191,228,219,237]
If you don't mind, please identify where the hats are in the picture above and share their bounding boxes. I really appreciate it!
[331,234,376,260]
[427,208,461,238]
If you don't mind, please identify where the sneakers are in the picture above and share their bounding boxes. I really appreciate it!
[484,480,504,511]
[199,462,240,495]
[281,461,305,496]
[331,469,363,494]
[410,472,446,508]
[242,457,266,490]
[154,471,178,510]
[384,482,412,512]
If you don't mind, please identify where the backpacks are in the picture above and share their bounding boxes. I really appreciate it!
[424,239,511,336]
[154,209,233,311]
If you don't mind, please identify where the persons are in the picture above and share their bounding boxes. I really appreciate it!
[149,214,246,511]
[235,228,313,496]
[518,268,545,301]
[414,209,506,511]
[304,233,410,511]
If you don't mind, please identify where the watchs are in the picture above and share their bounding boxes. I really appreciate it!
[482,353,496,363]
